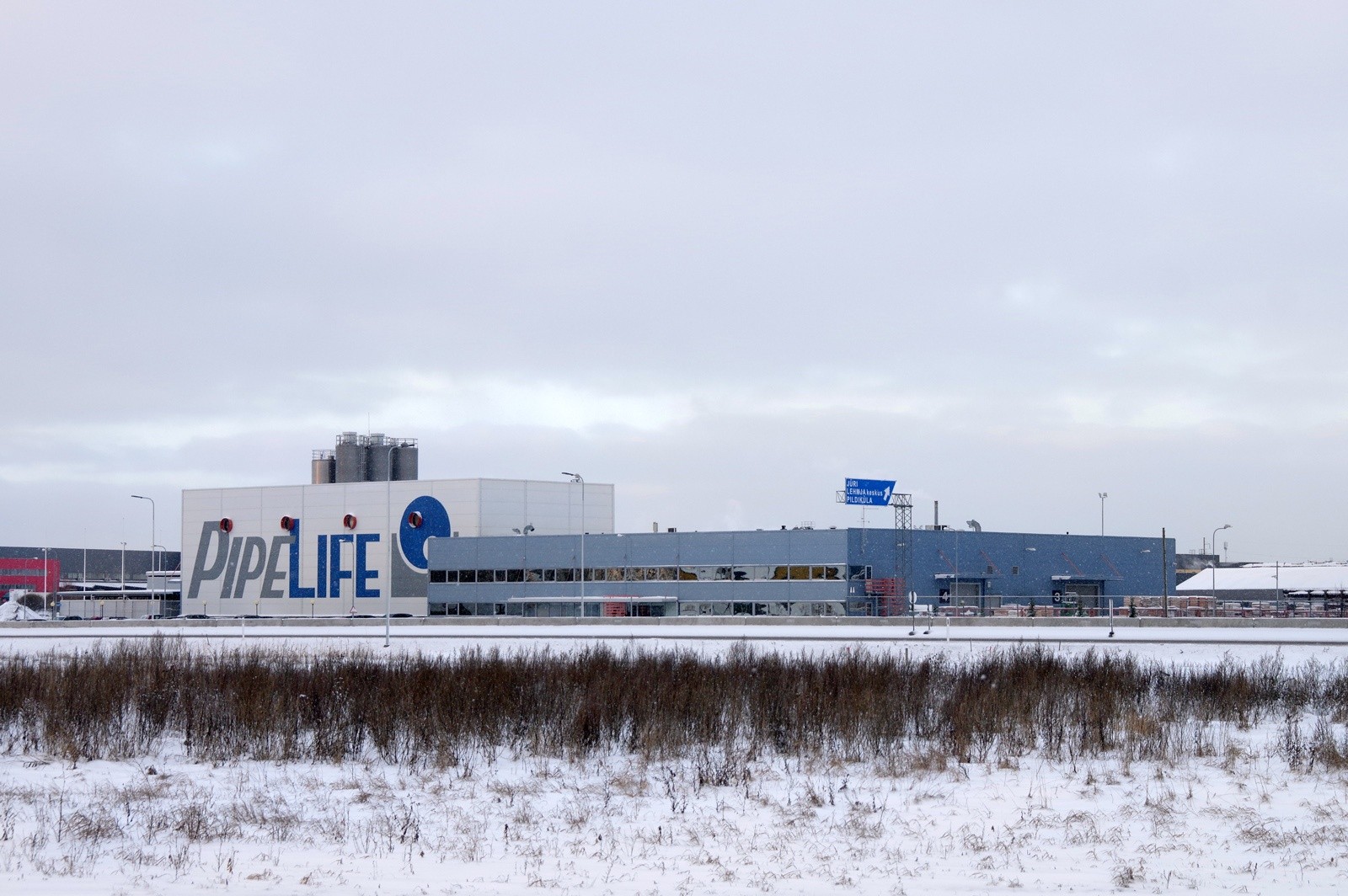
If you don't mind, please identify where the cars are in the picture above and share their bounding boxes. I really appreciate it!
[57,613,209,621]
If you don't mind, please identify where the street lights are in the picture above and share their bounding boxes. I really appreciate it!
[153,545,166,619]
[385,442,415,647]
[948,527,958,617]
[42,548,47,611]
[120,542,127,590]
[24,557,38,619]
[130,494,155,620]
[1212,524,1232,617]
[561,471,585,617]
[1098,492,1108,536]
